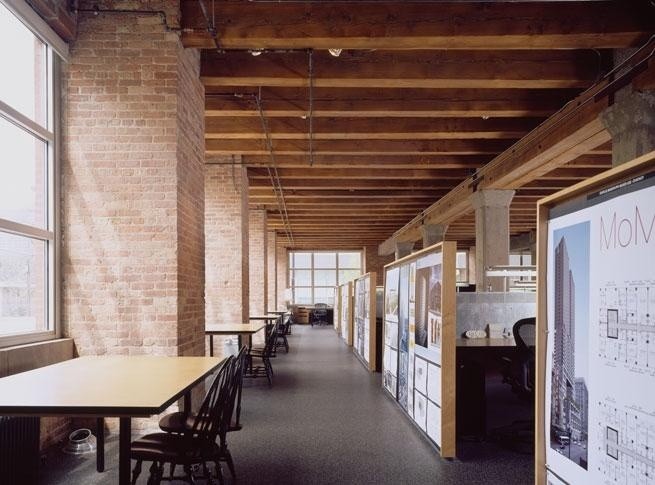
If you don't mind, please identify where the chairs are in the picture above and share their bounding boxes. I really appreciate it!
[491,317,535,445]
